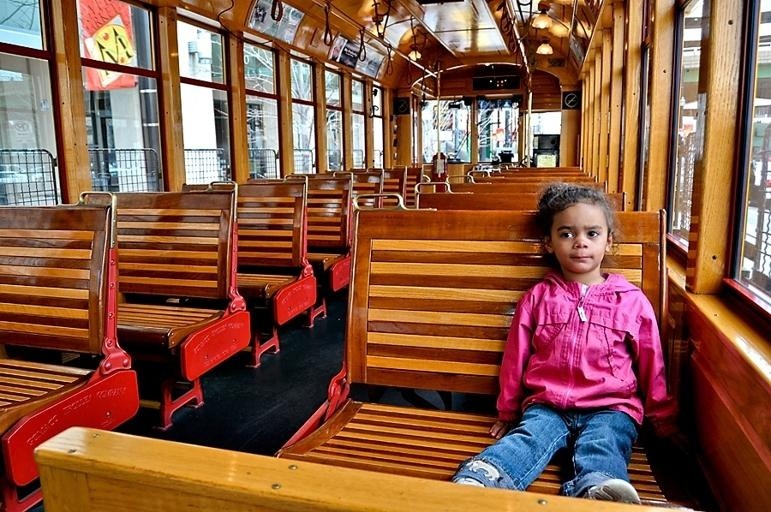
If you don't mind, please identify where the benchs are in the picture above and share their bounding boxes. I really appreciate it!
[414,163,626,211]
[84,181,251,431]
[384,165,407,208]
[273,194,669,508]
[307,172,354,328]
[182,175,317,368]
[407,163,422,209]
[33,426,681,511]
[354,168,384,208]
[0,191,140,511]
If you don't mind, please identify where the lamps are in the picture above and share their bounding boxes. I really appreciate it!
[408,47,421,61]
[532,1,552,30]
[369,22,384,38]
[536,37,553,55]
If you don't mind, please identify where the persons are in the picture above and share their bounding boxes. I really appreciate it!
[450,183,694,503]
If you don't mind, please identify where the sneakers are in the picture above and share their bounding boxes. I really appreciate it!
[582,479,642,505]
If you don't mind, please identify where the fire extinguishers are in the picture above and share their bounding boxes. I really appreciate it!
[433,152,449,193]
